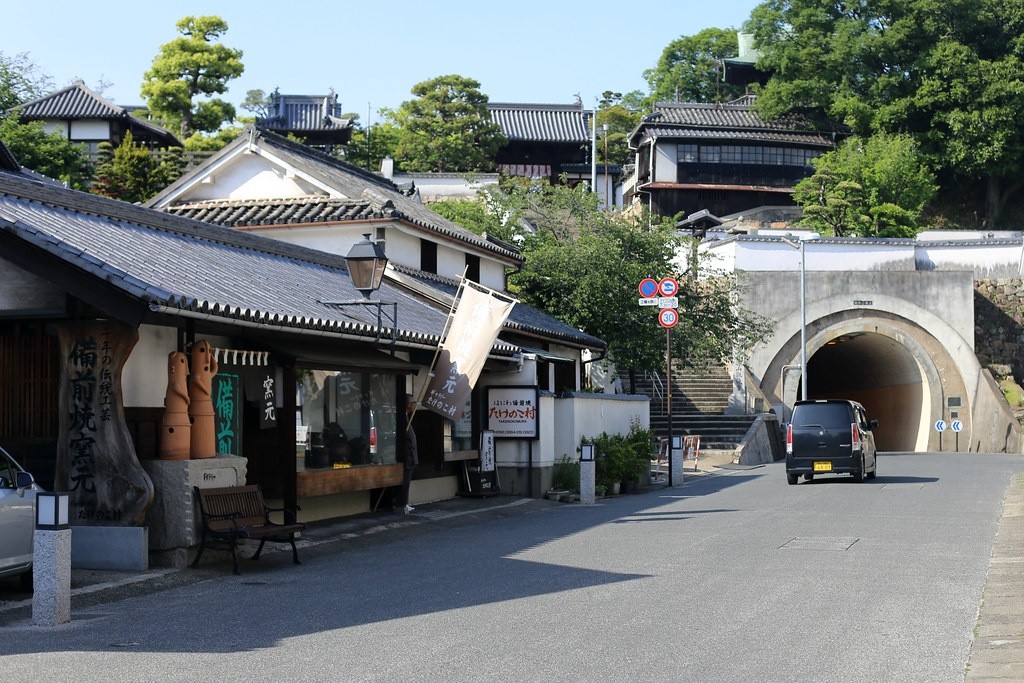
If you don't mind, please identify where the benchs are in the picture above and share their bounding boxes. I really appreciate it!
[190,483,307,574]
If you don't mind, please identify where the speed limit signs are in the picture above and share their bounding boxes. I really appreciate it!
[659,309,679,328]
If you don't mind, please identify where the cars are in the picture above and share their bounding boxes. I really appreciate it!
[0,445,49,578]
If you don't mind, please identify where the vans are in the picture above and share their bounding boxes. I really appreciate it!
[780,399,879,485]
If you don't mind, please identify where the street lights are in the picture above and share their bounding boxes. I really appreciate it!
[798,232,821,403]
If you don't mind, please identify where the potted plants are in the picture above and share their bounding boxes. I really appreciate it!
[546,415,656,501]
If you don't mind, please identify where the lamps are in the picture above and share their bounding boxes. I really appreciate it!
[322,230,398,346]
[36,491,70,530]
[672,435,682,450]
[581,444,594,460]
